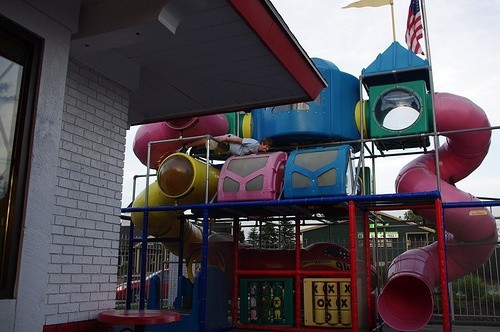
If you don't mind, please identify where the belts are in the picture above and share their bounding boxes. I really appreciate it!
[226,135,230,152]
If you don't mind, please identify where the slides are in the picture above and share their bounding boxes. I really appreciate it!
[377,92,499,332]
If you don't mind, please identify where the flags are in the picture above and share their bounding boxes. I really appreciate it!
[405,0,425,55]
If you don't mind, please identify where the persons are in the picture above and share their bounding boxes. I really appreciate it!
[177,134,272,155]
[448,176,456,187]
